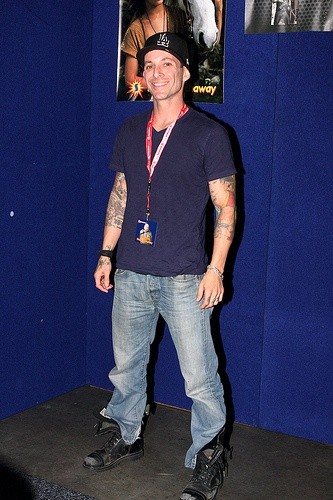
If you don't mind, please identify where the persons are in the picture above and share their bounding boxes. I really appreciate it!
[82,32,237,500]
[140,224,152,242]
[120,0,222,92]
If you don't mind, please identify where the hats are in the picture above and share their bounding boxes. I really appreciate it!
[136,32,191,70]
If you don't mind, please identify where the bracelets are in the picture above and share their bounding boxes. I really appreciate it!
[100,250,113,258]
[207,265,223,280]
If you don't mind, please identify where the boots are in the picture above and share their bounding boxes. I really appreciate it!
[178,424,229,500]
[82,403,151,472]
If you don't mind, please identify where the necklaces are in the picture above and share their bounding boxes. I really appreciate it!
[146,9,166,34]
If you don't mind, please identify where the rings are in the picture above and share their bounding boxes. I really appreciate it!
[215,296,219,301]
[220,294,223,298]
[205,297,209,302]
[209,298,215,304]
